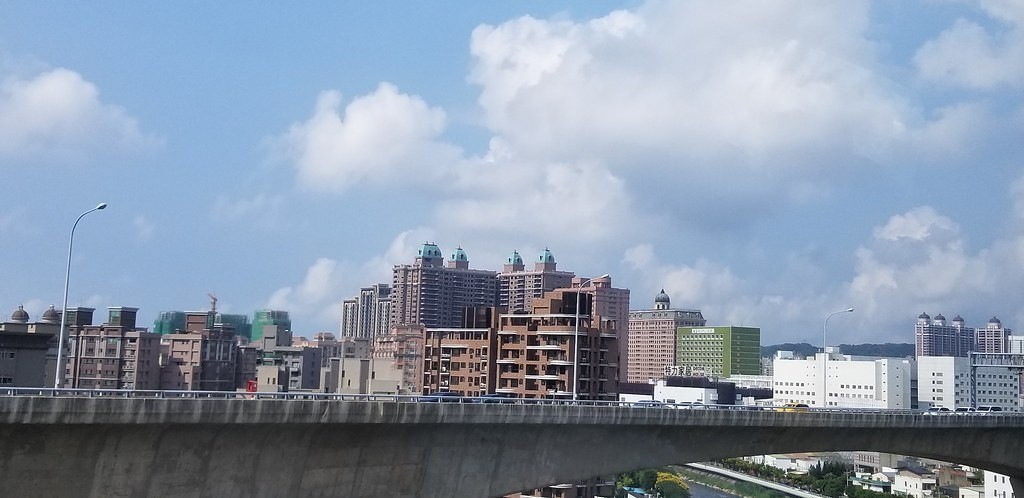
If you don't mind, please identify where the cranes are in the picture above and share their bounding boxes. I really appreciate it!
[207,292,218,313]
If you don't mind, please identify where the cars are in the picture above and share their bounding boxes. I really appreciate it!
[476,393,515,404]
[975,406,1003,416]
[420,391,473,403]
[923,407,950,416]
[953,407,977,416]
[676,401,706,409]
[633,399,664,407]
[734,406,764,411]
[777,403,810,413]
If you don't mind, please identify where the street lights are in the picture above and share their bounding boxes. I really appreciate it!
[823,307,855,412]
[52,201,107,396]
[572,273,610,405]
